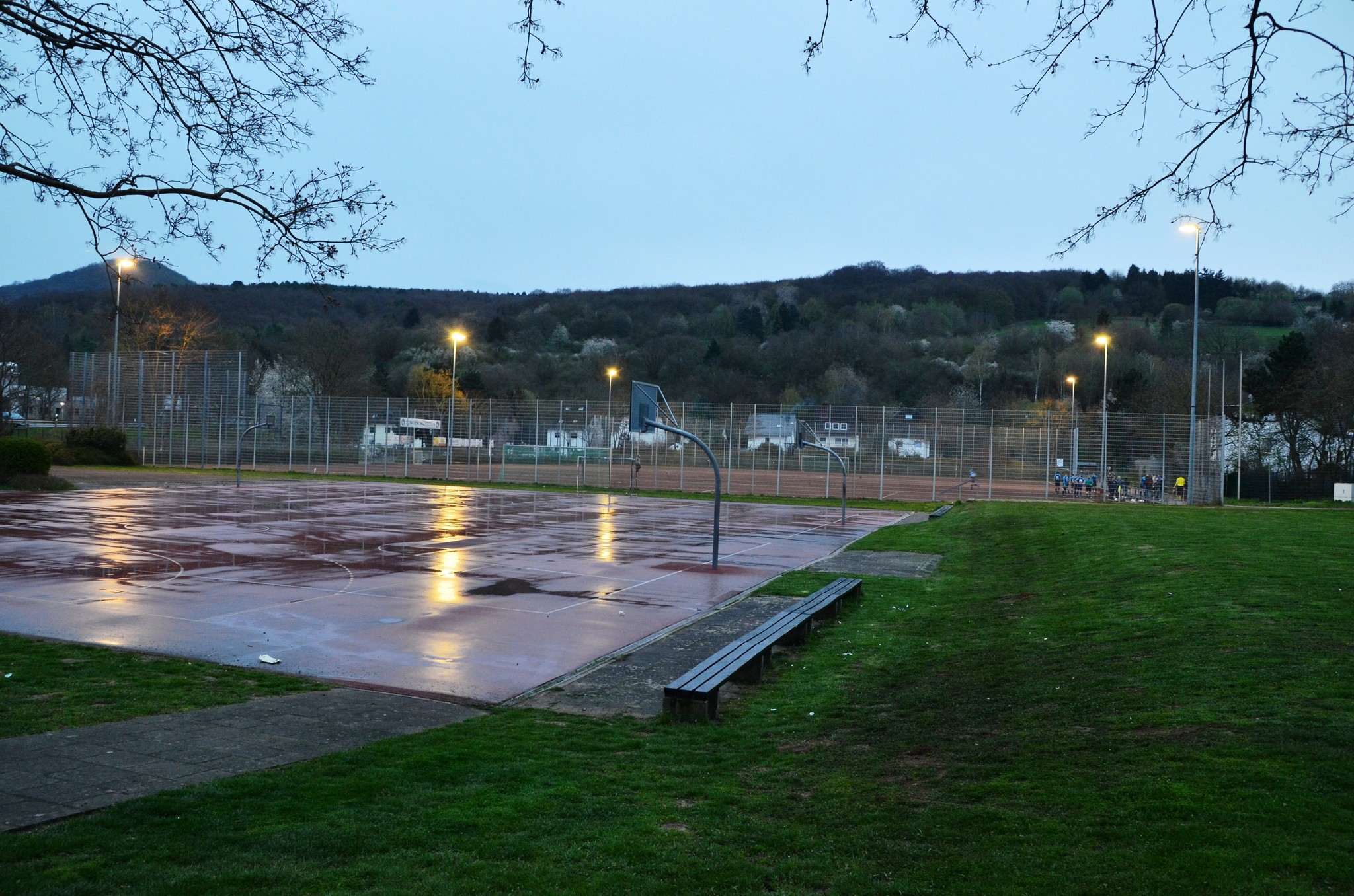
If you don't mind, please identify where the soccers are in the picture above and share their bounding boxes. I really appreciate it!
[1138,499,1144,503]
[1131,499,1134,501]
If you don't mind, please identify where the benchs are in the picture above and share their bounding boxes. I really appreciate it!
[662,576,863,722]
[927,505,954,520]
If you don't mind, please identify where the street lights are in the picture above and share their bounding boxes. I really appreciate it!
[606,365,619,461]
[449,327,470,464]
[1064,375,1077,480]
[1096,335,1112,488]
[110,253,137,426]
[1178,221,1204,508]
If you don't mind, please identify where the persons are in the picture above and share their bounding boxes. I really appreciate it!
[1172,475,1186,501]
[1053,465,1166,502]
[968,469,981,492]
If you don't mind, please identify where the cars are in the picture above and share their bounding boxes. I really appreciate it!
[2,411,27,427]
